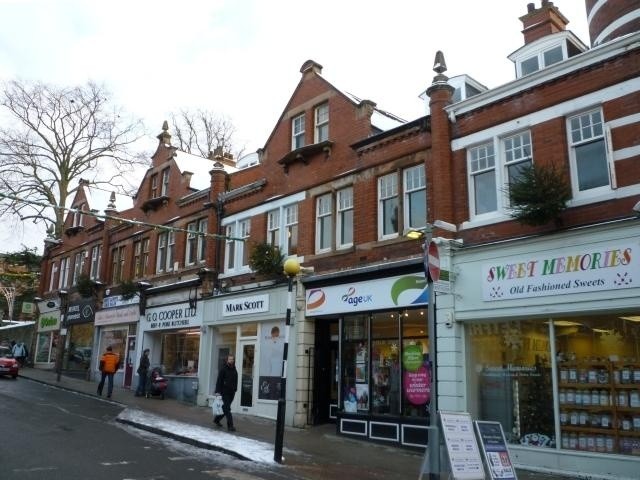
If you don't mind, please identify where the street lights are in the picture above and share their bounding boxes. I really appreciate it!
[272,257,301,464]
[404,219,458,479]
[33,291,69,382]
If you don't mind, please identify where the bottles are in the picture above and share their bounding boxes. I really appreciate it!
[558,352,640,455]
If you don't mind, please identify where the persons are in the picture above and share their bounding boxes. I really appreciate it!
[0,337,29,368]
[213,352,239,433]
[260,327,283,376]
[133,345,149,399]
[97,346,119,399]
[154,371,168,388]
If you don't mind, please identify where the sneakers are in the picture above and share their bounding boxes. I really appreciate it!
[214,420,223,427]
[228,427,236,431]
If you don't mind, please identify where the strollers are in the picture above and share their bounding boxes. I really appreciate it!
[146,366,169,400]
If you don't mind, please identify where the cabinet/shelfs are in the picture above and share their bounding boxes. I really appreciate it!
[557,360,640,461]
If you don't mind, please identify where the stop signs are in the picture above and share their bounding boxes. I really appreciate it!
[424,241,442,284]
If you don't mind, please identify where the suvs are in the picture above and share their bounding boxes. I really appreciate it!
[0,343,18,380]
